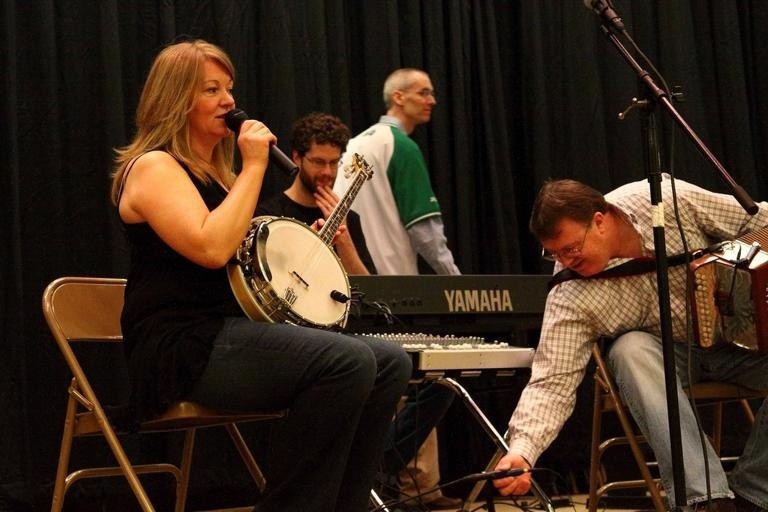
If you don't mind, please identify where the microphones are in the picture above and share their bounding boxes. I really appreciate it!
[583,0,624,33]
[331,290,347,304]
[224,108,299,179]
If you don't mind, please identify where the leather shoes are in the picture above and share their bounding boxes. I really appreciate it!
[410,496,464,510]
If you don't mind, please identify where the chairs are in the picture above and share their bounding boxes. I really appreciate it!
[588,342,768,512]
[41,276,281,512]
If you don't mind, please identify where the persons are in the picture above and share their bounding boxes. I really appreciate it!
[489,170,767,511]
[260,108,463,507]
[323,63,464,509]
[108,36,416,510]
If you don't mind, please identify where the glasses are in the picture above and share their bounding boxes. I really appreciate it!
[305,154,343,169]
[542,221,592,262]
